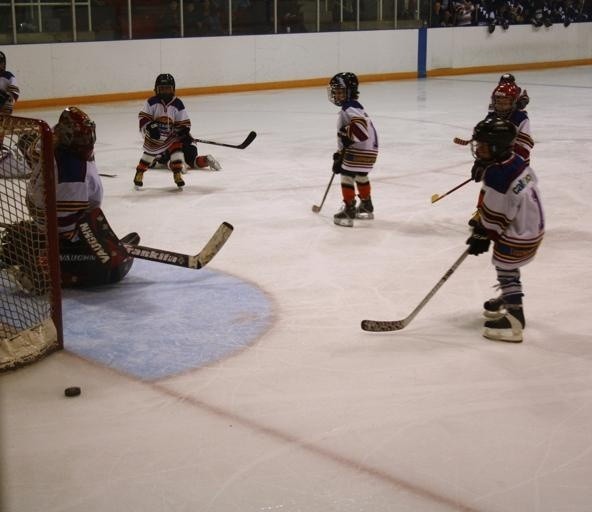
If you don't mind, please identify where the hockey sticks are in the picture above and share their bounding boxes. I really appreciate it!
[432,179,475,203]
[121,222,233,269]
[193,132,256,149]
[454,138,473,145]
[361,249,469,331]
[312,172,336,212]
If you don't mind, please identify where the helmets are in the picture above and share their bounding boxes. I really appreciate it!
[326,71,360,106]
[154,73,175,97]
[492,84,521,113]
[471,116,517,162]
[0,51,6,72]
[53,107,97,159]
[500,72,516,84]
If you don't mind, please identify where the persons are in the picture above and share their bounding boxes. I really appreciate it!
[134,74,191,190]
[0,52,20,142]
[471,74,534,181]
[148,134,220,174]
[0,107,140,296]
[0,0,305,36]
[0,132,40,179]
[466,116,545,342]
[327,72,379,226]
[428,0,592,31]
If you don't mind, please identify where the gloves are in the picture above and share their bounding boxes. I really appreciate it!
[332,152,344,174]
[145,123,161,140]
[467,231,490,256]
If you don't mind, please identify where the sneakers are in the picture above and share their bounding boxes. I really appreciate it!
[206,154,222,171]
[468,210,482,227]
[484,307,525,331]
[334,205,356,219]
[173,171,185,186]
[356,200,374,213]
[484,294,522,312]
[133,168,144,186]
